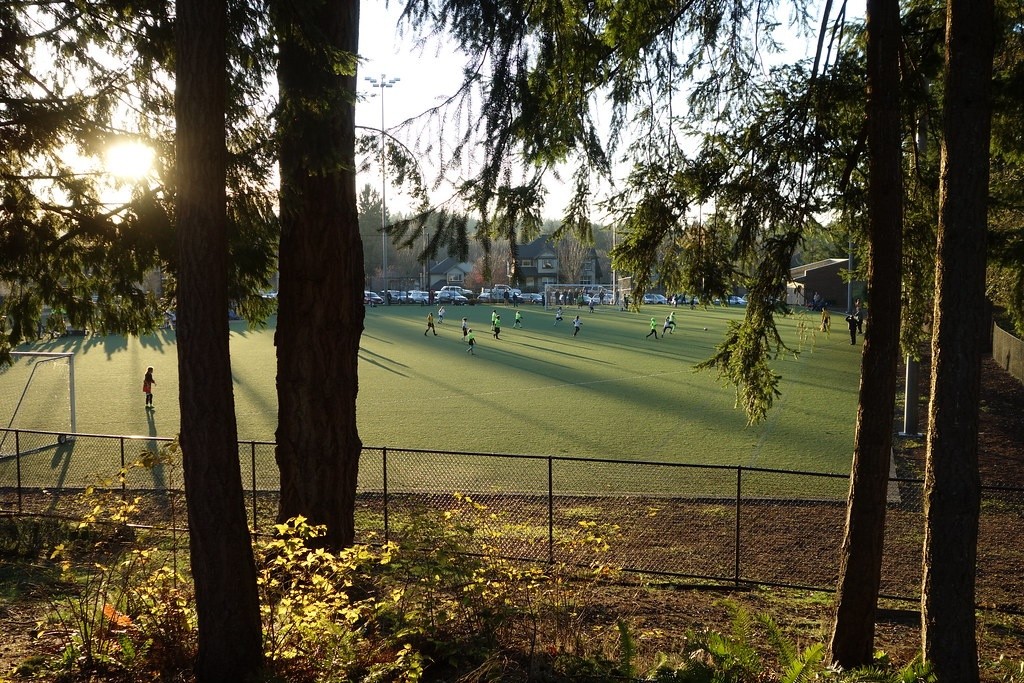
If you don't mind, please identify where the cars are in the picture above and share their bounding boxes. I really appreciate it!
[476,294,496,303]
[643,293,668,305]
[377,290,470,307]
[493,283,614,306]
[365,290,385,306]
[672,294,748,306]
[441,286,473,296]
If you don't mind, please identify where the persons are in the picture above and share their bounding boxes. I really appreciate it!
[846,315,858,345]
[573,315,584,337]
[554,307,563,326]
[670,311,676,331]
[666,290,695,310]
[821,307,831,332]
[542,288,629,313]
[513,311,523,328]
[34,314,60,339]
[811,291,828,311]
[466,328,476,355]
[462,317,468,341]
[424,312,438,336]
[438,306,445,324]
[662,316,672,338]
[646,318,659,339]
[492,309,498,331]
[387,289,519,308]
[854,298,863,333]
[142,367,156,408]
[494,314,501,339]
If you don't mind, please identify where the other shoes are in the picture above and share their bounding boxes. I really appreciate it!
[150,405,155,408]
[145,405,149,408]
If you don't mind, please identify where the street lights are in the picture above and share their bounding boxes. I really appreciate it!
[365,71,400,307]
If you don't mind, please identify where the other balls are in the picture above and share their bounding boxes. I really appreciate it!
[704,327,707,331]
